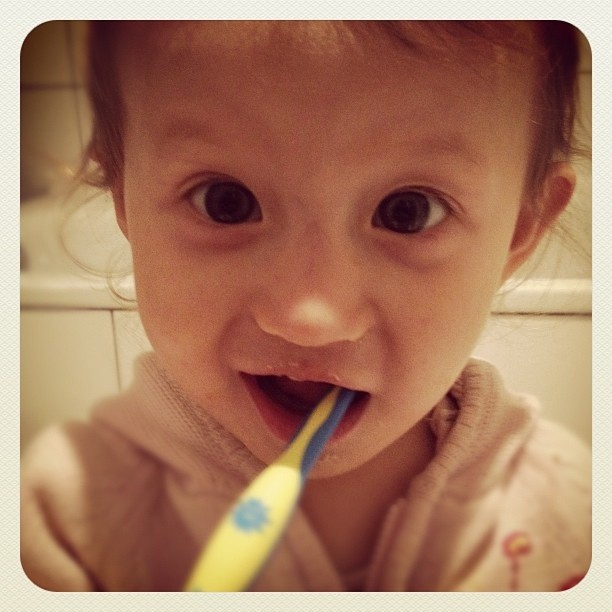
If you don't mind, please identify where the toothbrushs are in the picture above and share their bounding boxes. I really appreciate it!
[174,363,361,585]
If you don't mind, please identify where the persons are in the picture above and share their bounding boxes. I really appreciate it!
[19,21,592,592]
